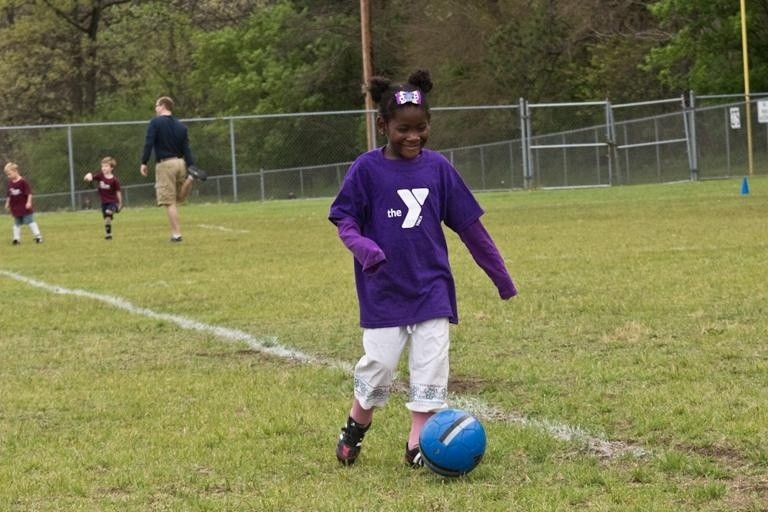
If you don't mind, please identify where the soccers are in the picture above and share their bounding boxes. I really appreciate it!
[419,409,486,477]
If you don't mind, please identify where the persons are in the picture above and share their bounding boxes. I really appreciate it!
[84,157,122,240]
[328,70,518,469]
[3,161,43,245]
[139,96,207,243]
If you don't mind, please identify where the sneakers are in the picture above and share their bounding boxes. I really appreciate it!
[337,415,371,465]
[403,441,424,471]
[189,166,206,181]
[170,233,182,242]
